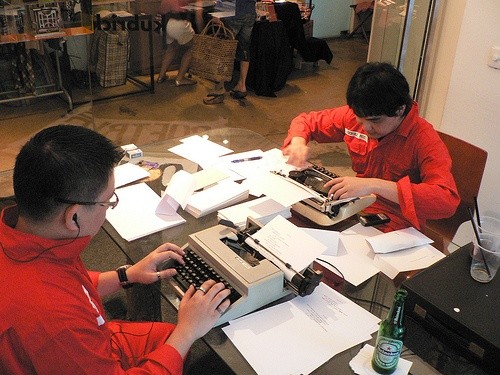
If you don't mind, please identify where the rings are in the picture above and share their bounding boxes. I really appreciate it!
[199,286,207,294]
[216,307,223,315]
[156,272,161,280]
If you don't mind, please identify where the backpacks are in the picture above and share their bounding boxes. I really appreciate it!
[0,42,60,107]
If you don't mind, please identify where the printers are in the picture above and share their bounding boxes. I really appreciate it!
[269,162,377,226]
[158,217,324,328]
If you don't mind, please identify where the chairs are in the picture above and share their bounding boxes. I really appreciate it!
[393,131,488,288]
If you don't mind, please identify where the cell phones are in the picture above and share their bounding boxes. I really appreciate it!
[359,213,390,227]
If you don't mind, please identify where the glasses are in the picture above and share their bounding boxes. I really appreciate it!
[56,192,119,209]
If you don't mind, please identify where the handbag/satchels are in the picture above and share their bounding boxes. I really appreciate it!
[90,22,132,89]
[187,20,239,82]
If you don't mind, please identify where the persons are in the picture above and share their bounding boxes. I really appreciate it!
[0,125,230,375]
[201,0,256,96]
[156,0,197,85]
[280,62,460,292]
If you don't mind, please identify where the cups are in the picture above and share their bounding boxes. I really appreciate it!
[473,216,500,246]
[470,233,500,283]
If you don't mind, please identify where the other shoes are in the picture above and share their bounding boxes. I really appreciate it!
[176,79,194,87]
[157,75,168,84]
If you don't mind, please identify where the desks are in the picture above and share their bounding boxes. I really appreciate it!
[97,124,460,375]
[0,27,93,114]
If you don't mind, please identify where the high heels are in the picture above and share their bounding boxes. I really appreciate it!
[229,89,247,98]
[203,94,224,105]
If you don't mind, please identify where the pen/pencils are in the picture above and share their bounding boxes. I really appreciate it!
[231,157,262,163]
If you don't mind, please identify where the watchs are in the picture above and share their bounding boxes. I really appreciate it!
[116,265,133,289]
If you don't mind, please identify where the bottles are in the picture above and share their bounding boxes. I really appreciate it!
[370,288,408,375]
